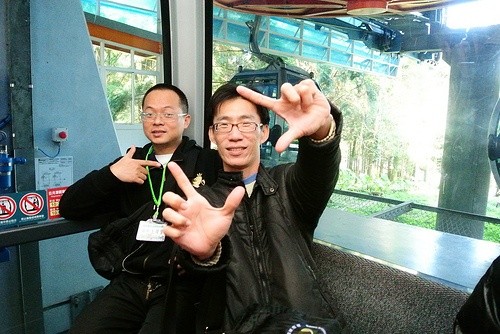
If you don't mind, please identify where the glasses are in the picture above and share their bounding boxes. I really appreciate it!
[139,112,187,122]
[212,121,263,134]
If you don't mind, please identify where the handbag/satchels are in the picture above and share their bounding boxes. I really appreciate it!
[453,256,500,334]
[88,202,154,280]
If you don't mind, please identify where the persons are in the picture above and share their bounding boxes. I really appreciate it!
[59,83,227,334]
[162,78,344,334]
